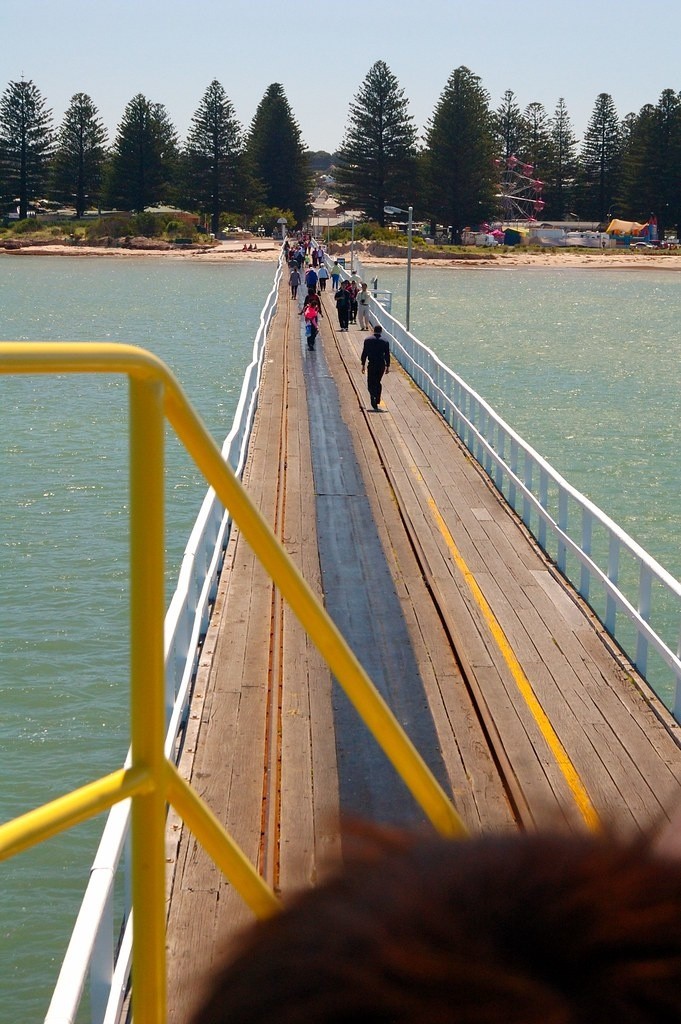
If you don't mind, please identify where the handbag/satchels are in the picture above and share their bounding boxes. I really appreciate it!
[305,320,312,337]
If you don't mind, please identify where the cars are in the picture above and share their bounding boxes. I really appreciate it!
[628,242,652,251]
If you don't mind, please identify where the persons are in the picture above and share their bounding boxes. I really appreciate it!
[283,234,362,301]
[333,279,371,332]
[298,288,323,351]
[360,324,390,411]
[242,242,257,252]
[187,831,681,1023]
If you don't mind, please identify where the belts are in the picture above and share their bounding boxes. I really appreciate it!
[360,304,368,306]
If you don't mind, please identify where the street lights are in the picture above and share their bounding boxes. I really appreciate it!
[382,205,413,331]
[340,212,355,276]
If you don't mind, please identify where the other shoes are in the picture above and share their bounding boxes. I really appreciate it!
[345,328,348,331]
[340,328,344,331]
